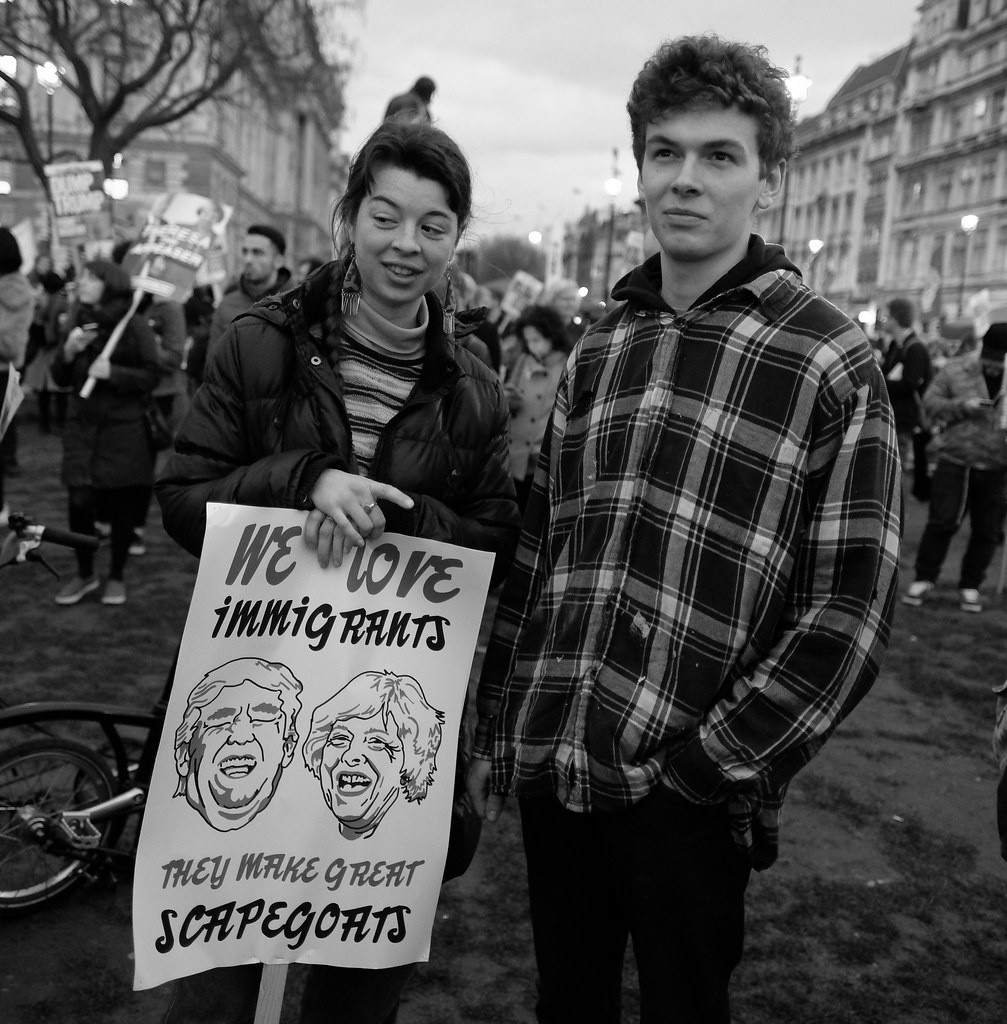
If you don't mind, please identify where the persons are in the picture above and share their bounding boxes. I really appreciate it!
[467,35,904,1024]
[154,78,518,1023]
[0,222,1006,615]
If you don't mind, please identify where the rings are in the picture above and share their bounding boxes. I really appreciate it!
[364,502,378,513]
[327,517,333,523]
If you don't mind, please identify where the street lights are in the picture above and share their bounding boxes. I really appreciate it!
[958,214,979,320]
[809,238,824,296]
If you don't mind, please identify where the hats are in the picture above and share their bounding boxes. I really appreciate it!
[981,322,1007,360]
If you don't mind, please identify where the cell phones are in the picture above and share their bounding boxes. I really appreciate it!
[980,394,1002,409]
[503,383,525,401]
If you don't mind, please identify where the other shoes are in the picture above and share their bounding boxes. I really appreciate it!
[53,575,98,603]
[958,587,984,611]
[101,580,128,604]
[902,580,935,606]
[93,520,112,535]
[128,536,145,554]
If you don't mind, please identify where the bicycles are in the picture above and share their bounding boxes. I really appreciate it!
[0,505,168,916]
